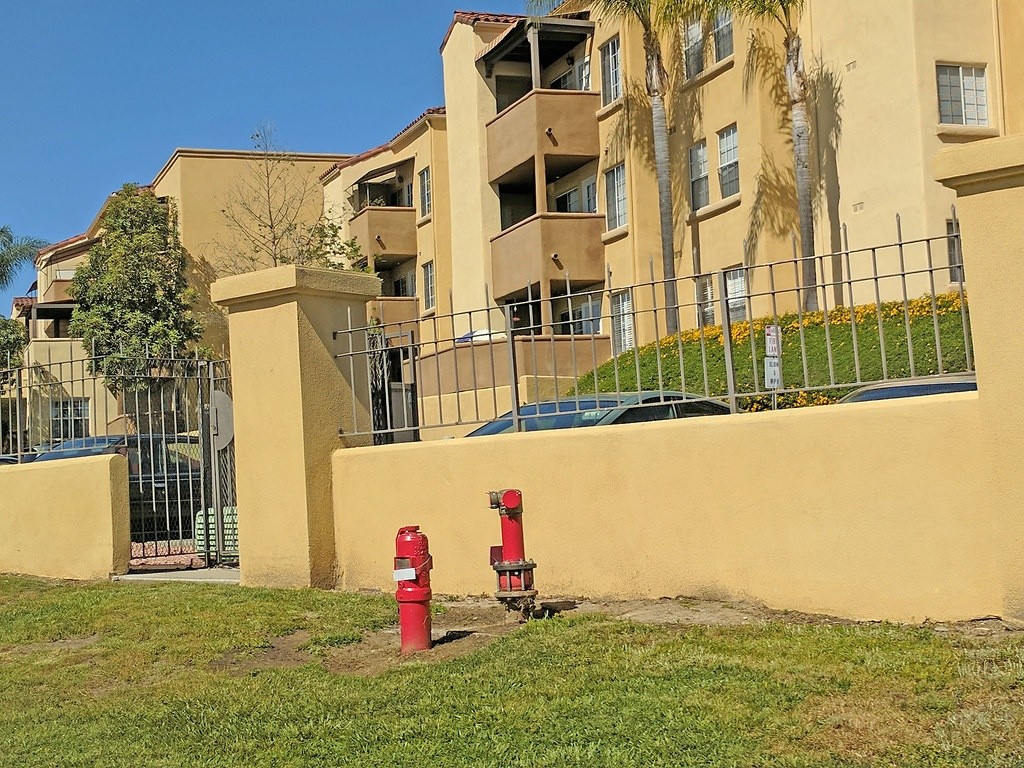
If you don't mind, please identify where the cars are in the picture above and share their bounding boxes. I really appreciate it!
[29,433,199,542]
[460,391,747,438]
[831,375,978,403]
